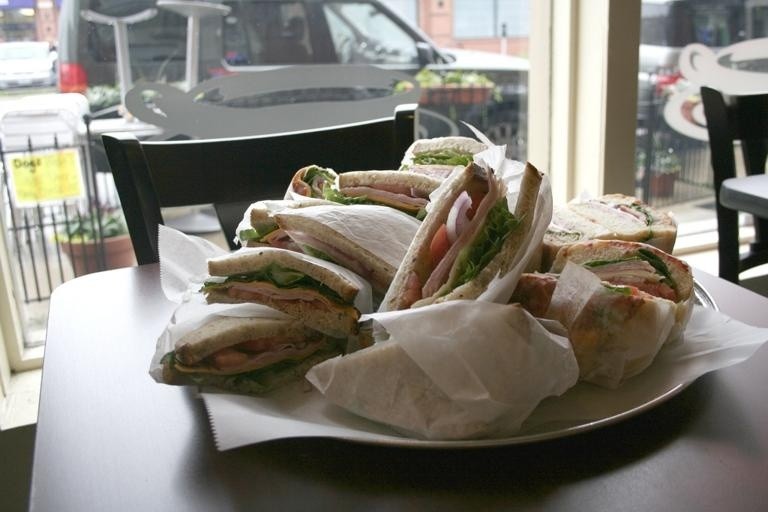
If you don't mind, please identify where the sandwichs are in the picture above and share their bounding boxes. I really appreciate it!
[173,136,695,439]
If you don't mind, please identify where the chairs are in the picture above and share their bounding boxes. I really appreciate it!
[99,102,422,268]
[700,81,768,283]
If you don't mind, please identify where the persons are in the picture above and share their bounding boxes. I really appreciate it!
[285,14,308,55]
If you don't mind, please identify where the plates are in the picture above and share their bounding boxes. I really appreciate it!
[238,278,722,448]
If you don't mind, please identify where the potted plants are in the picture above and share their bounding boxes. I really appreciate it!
[413,66,498,107]
[53,212,134,278]
[643,149,682,197]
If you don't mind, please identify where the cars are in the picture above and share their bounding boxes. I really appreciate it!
[47,1,528,147]
[638,0,768,151]
